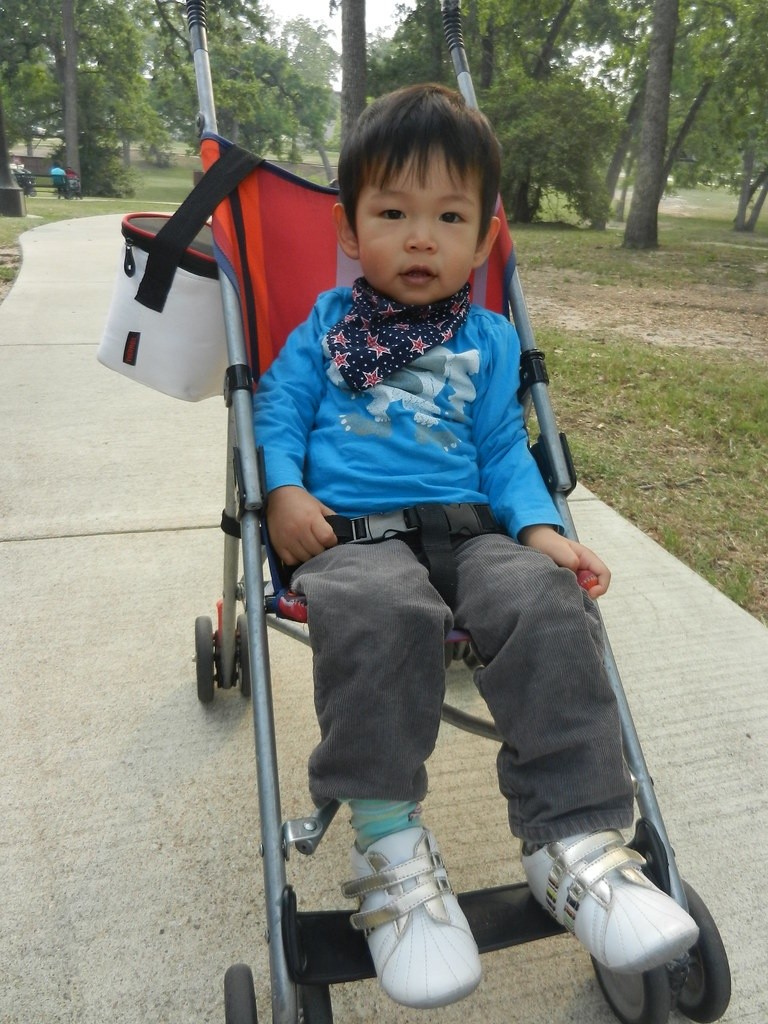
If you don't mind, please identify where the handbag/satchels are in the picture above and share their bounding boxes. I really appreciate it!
[96,143,266,401]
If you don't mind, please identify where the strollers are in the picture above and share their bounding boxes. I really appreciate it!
[107,1,733,1024]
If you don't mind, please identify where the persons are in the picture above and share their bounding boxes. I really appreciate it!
[52,160,68,199]
[252,84,700,1010]
[10,159,36,196]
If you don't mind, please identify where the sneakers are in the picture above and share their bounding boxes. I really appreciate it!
[521,828,699,975]
[340,826,481,1010]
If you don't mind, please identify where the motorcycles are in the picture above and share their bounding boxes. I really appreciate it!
[12,168,37,198]
[56,177,83,200]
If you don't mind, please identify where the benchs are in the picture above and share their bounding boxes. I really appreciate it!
[16,173,68,199]
